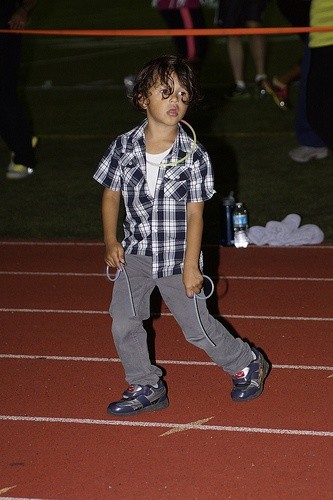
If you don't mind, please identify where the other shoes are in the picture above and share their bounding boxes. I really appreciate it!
[6,162,31,180]
[289,145,329,162]
[224,83,251,101]
[253,78,267,99]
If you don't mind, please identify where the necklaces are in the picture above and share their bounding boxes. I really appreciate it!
[144,119,198,167]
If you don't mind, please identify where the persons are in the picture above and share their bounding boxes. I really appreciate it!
[93,54,271,415]
[153,0,333,162]
[0,0,39,180]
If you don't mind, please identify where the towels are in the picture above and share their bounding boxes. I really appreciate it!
[243,214,326,247]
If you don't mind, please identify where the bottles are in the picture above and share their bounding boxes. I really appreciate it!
[233,202,249,248]
[219,197,235,247]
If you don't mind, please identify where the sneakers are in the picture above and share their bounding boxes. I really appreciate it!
[230,347,269,401]
[264,77,288,111]
[107,378,169,416]
